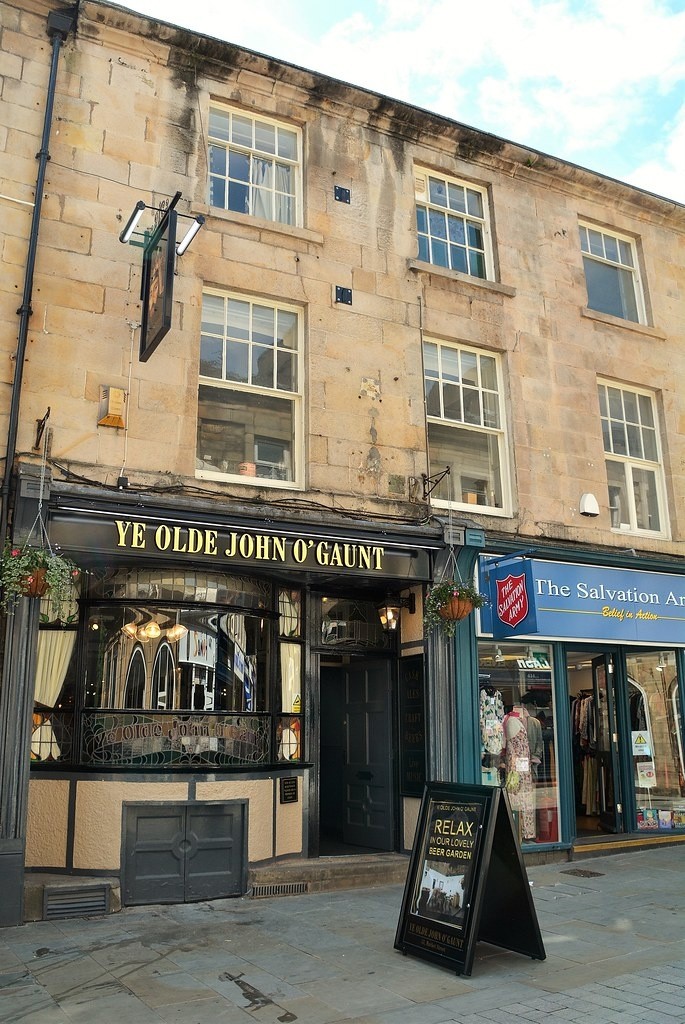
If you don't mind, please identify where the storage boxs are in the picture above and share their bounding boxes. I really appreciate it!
[463,493,478,504]
[637,809,685,830]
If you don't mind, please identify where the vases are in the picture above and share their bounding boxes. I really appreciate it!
[17,567,49,597]
[437,596,474,620]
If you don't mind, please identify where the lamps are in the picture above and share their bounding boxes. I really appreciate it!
[575,663,583,670]
[524,650,539,662]
[136,627,150,642]
[658,656,667,667]
[166,628,177,643]
[494,649,505,662]
[376,591,416,641]
[121,622,138,640]
[172,625,189,641]
[656,658,662,671]
[579,493,601,517]
[146,622,161,638]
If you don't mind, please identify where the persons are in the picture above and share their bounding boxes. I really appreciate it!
[504,704,544,843]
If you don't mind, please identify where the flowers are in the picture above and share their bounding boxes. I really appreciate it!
[420,574,485,646]
[0,536,95,637]
